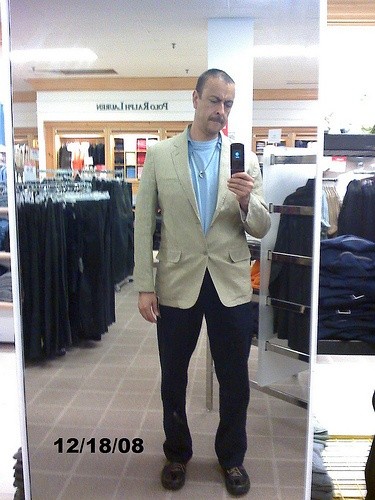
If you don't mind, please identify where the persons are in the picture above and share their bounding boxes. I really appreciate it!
[132,68,271,498]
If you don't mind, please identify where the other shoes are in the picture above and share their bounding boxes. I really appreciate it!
[218,458,250,496]
[161,459,186,490]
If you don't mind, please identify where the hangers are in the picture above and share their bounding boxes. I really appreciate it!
[15,170,125,204]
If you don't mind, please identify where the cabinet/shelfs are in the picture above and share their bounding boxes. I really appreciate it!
[108,128,163,212]
[254,135,288,180]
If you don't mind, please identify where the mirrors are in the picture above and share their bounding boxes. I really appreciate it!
[0,0,327,500]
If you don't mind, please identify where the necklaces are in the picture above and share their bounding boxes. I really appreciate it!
[192,135,221,179]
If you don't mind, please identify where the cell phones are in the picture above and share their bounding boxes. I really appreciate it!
[230,143,245,179]
